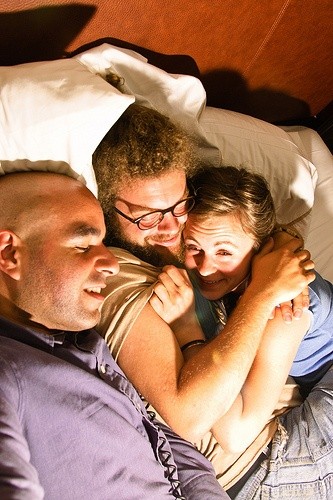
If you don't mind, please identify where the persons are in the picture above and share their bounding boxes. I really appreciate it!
[147,165,333,394]
[91,105,333,500]
[0,170,231,500]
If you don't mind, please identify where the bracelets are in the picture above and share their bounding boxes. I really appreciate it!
[180,339,207,353]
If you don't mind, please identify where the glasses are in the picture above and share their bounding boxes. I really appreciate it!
[112,175,198,231]
